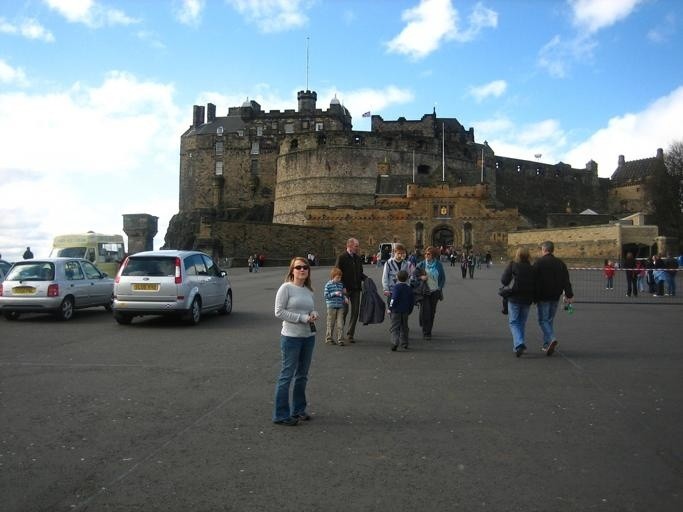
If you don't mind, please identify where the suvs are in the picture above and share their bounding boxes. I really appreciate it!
[110,249,233,325]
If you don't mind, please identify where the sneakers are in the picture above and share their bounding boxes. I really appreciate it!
[545,338,558,357]
[348,337,355,343]
[338,341,345,346]
[326,340,336,344]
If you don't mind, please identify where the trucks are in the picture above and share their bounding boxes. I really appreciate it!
[379,242,401,264]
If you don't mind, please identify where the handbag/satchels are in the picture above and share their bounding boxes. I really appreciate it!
[498,262,519,298]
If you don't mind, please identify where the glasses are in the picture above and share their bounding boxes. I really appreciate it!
[293,265,308,270]
[425,253,430,255]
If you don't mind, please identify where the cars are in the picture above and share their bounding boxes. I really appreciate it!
[0,257,115,321]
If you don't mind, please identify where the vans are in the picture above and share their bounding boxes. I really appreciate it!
[48,231,126,278]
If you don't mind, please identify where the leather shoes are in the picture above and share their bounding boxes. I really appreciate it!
[294,413,310,420]
[275,416,299,426]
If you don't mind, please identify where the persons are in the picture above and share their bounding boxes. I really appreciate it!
[477,252,482,270]
[468,251,475,279]
[486,251,492,267]
[654,270,669,296]
[388,270,414,352]
[23,247,32,259]
[382,245,416,307]
[248,256,253,272]
[461,260,467,278]
[656,256,665,268]
[335,238,368,344]
[271,257,319,426]
[605,259,617,290]
[324,268,349,346]
[532,241,573,356]
[414,246,446,342]
[440,245,457,267]
[665,253,679,295]
[625,252,638,296]
[501,246,532,358]
[369,247,381,267]
[636,261,646,292]
[652,255,657,264]
[253,254,260,273]
